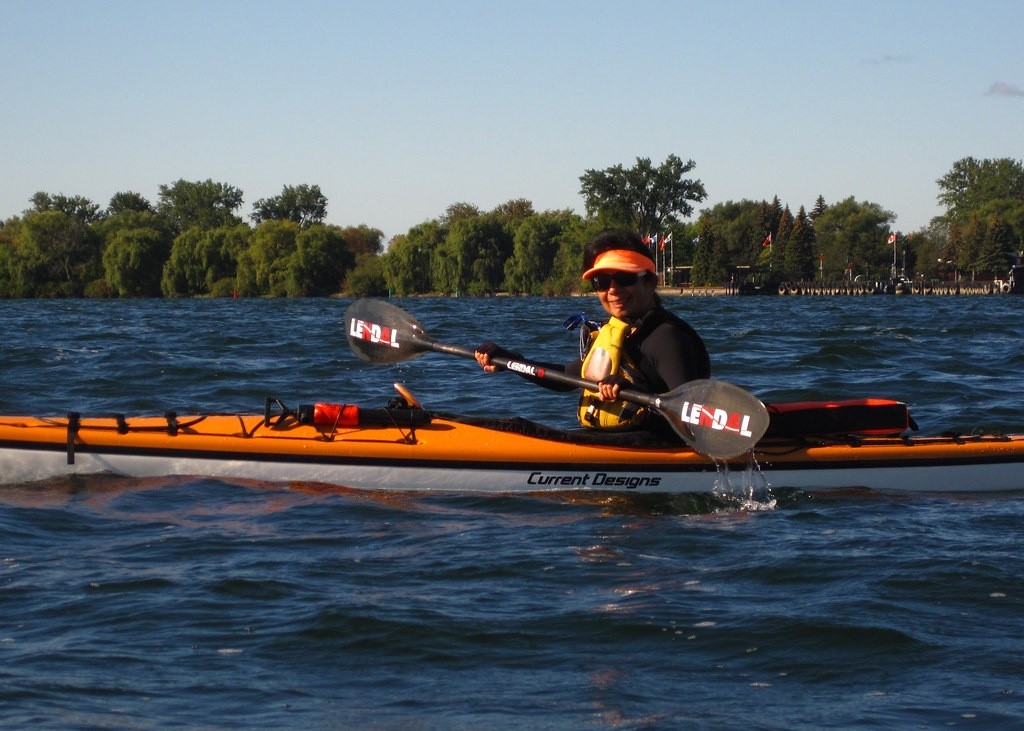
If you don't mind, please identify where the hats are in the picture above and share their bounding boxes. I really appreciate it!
[583,250,655,280]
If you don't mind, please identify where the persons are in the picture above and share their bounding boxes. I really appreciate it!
[473,229,712,431]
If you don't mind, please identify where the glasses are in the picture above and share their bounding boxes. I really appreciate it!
[592,272,646,290]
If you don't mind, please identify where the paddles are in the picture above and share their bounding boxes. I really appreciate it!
[343,297,769,459]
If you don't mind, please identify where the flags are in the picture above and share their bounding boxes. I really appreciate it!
[762,233,772,246]
[888,232,896,244]
[642,231,672,250]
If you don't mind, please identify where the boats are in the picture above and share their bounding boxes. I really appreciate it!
[0,397,1023,506]
